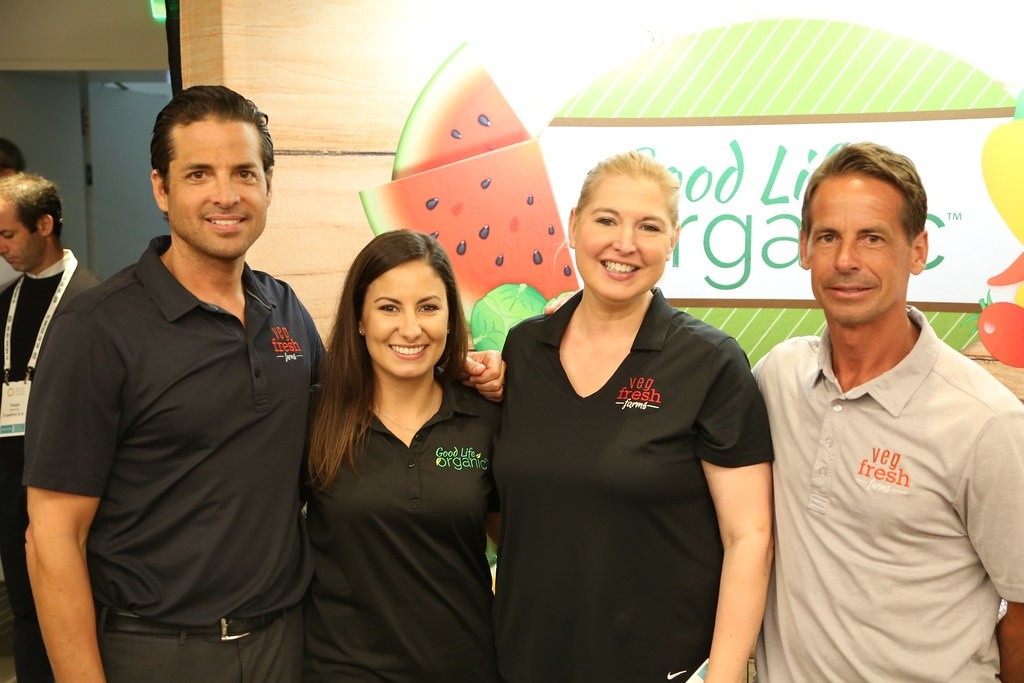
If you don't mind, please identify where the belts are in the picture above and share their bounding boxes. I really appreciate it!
[105,607,292,641]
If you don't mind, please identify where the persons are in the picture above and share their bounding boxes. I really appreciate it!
[0,172,105,683]
[0,138,27,292]
[299,229,503,683]
[23,86,506,683]
[543,142,1024,683]
[489,152,773,683]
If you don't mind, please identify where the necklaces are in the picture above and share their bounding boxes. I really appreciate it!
[375,382,437,430]
